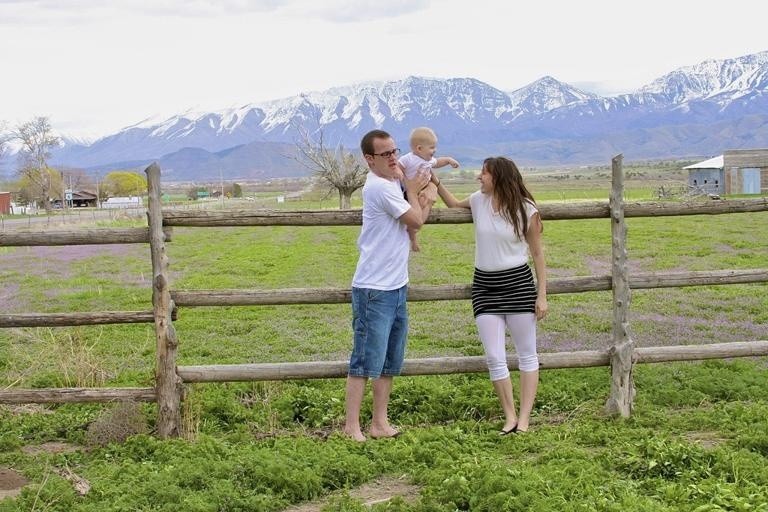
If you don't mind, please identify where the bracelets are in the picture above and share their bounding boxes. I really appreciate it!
[436,181,441,187]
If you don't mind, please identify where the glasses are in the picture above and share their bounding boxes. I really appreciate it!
[374,148,400,159]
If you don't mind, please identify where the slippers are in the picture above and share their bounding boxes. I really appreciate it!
[371,432,403,439]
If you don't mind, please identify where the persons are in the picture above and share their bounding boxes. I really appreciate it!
[394,126,460,253]
[339,129,435,442]
[426,155,551,438]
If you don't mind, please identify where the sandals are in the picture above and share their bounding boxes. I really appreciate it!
[499,424,527,436]
